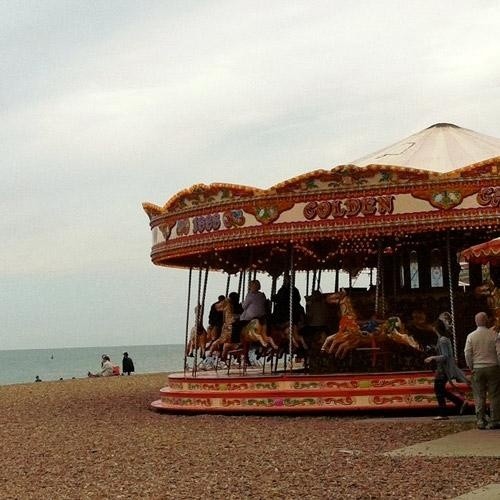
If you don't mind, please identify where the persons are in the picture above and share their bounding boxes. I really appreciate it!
[121,352,135,375]
[88,356,114,378]
[100,354,107,367]
[34,375,42,382]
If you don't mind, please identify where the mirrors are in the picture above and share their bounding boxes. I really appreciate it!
[399,249,490,289]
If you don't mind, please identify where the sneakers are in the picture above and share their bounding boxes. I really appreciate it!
[459,401,468,416]
[433,415,450,421]
[477,422,496,430]
